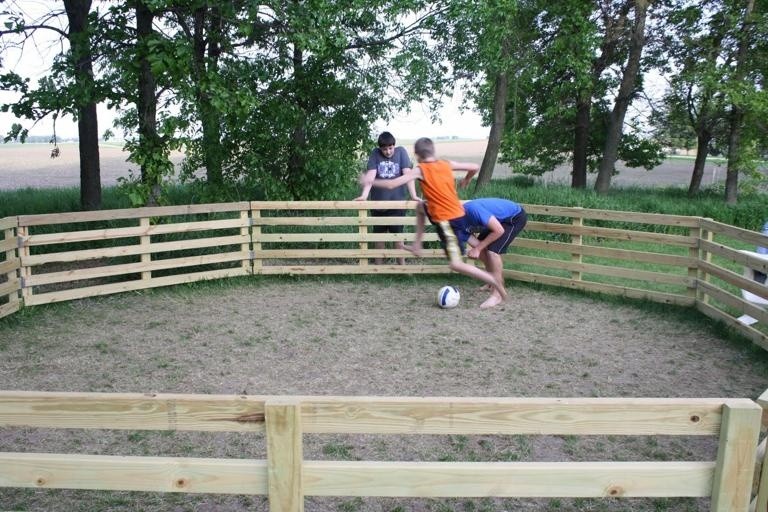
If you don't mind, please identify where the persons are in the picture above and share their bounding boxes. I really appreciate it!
[353,131,427,278]
[735,221,766,325]
[449,196,528,311]
[352,137,510,301]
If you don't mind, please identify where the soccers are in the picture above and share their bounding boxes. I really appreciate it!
[437,284,461,309]
